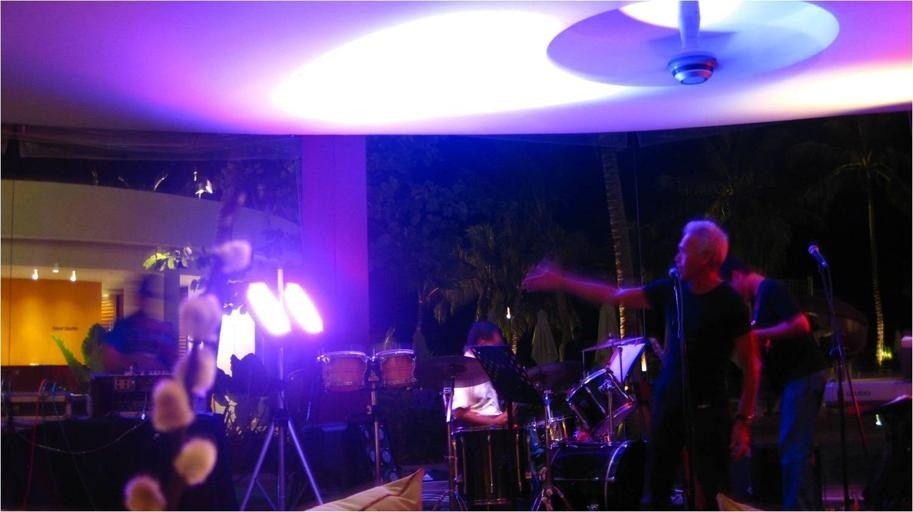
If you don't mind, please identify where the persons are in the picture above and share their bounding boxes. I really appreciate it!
[97,271,178,371]
[720,257,828,511]
[519,220,761,510]
[450,321,519,426]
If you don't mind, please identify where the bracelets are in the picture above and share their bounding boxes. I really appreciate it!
[735,415,753,428]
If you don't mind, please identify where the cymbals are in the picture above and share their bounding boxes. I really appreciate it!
[580,336,645,352]
[415,356,490,388]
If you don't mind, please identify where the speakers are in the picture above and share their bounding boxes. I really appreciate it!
[1,412,233,511]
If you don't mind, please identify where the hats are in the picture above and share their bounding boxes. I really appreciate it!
[139,273,175,303]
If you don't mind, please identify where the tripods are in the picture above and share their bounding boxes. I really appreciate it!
[530,403,572,511]
[239,350,326,510]
[429,405,468,511]
[806,299,876,511]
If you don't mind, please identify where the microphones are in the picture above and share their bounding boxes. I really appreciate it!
[667,266,686,330]
[807,241,830,270]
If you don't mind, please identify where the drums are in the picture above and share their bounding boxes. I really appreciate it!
[565,367,639,438]
[316,350,368,394]
[375,349,417,389]
[540,438,653,510]
[450,425,534,505]
[526,416,578,446]
